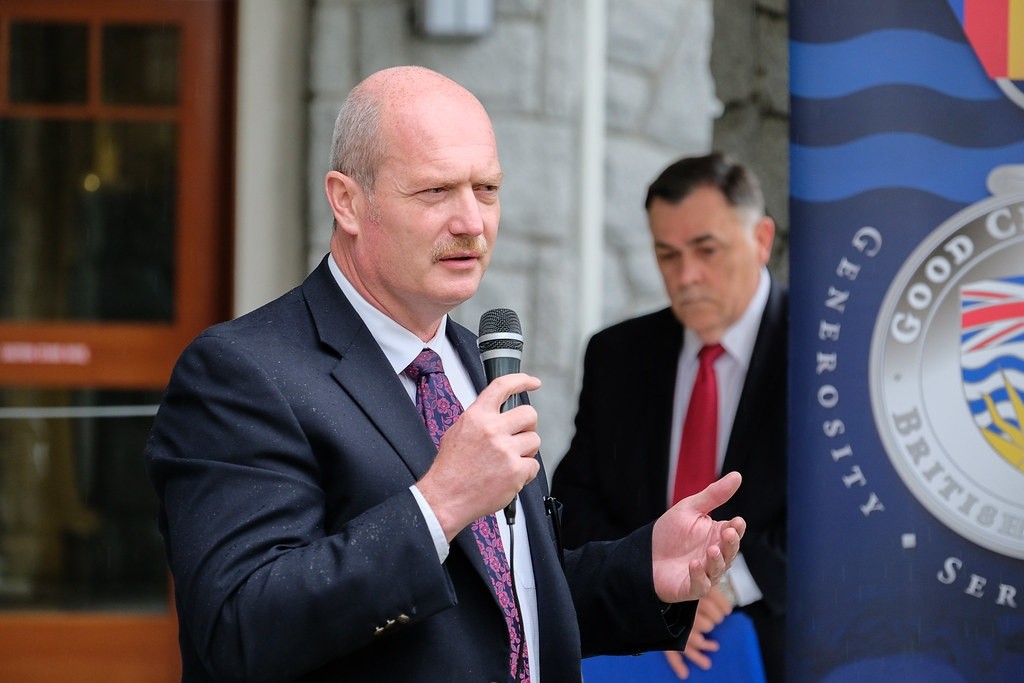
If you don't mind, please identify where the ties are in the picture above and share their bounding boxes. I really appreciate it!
[402,348,530,683]
[672,342,724,508]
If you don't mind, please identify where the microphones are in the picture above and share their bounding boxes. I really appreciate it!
[478,308,523,526]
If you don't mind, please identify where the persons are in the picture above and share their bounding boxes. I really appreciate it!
[148,62,747,683]
[549,152,789,681]
